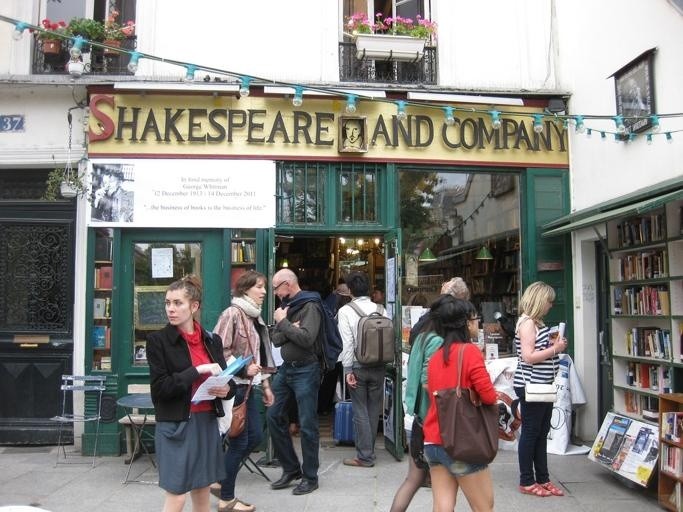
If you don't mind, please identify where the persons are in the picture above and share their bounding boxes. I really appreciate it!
[512,282,568,497]
[92,236,112,370]
[210,271,276,511]
[389,277,497,512]
[146,275,237,511]
[324,275,390,467]
[94,169,135,223]
[272,269,325,494]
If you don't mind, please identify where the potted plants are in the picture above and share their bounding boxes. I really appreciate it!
[66,17,104,53]
[40,153,96,207]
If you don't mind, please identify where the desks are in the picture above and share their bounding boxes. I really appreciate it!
[115,392,160,486]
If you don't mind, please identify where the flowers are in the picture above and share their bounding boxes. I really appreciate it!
[344,11,438,40]
[105,11,135,41]
[29,18,68,40]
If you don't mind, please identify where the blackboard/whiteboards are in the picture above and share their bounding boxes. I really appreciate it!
[134,285,171,331]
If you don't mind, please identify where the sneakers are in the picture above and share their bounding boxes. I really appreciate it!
[217,497,256,512]
[209,485,222,498]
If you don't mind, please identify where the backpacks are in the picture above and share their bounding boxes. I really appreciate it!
[346,301,395,365]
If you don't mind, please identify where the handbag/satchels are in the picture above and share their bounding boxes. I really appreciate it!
[524,381,557,403]
[227,400,247,439]
[410,415,428,469]
[217,395,235,436]
[432,345,499,465]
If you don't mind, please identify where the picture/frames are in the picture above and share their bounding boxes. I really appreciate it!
[338,115,369,153]
[614,52,655,141]
[490,174,515,198]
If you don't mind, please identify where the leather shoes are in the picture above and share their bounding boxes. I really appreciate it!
[343,458,362,466]
[292,479,318,495]
[271,468,303,488]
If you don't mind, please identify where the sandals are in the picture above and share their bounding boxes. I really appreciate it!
[540,482,563,496]
[520,482,551,497]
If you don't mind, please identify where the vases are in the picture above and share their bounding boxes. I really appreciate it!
[43,38,61,55]
[102,39,122,57]
[353,34,427,63]
[68,60,85,77]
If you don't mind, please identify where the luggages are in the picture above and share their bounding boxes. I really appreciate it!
[333,366,354,446]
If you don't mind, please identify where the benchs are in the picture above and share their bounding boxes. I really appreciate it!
[118,384,156,465]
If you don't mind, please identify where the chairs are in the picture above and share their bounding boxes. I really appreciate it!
[49,374,106,468]
[223,421,271,481]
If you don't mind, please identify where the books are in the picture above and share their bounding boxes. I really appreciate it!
[613,214,683,512]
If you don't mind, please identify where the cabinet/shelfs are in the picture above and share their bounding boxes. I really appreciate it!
[658,392,683,512]
[402,228,520,318]
[332,236,384,300]
[606,199,683,425]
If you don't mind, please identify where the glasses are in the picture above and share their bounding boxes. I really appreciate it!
[272,281,286,290]
[469,315,482,323]
